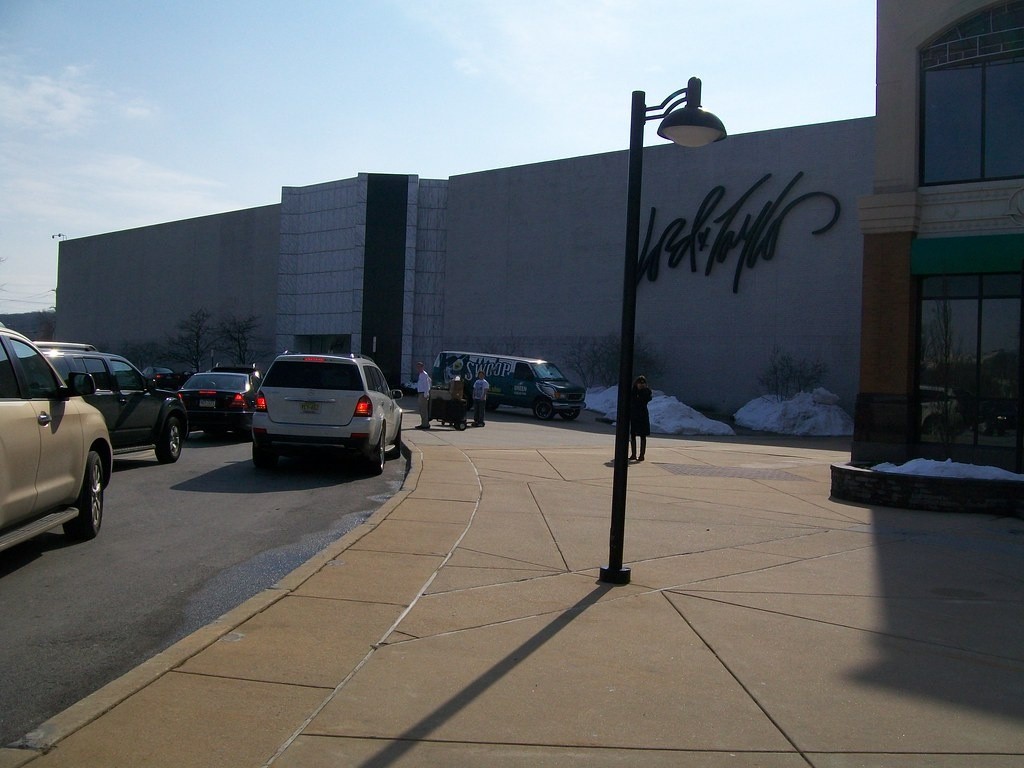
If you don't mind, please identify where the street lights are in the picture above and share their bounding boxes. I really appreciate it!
[592,77,730,584]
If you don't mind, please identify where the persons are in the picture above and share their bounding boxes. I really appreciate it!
[471,371,489,427]
[628,375,652,461]
[409,362,432,429]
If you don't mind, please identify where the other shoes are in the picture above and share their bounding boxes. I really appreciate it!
[637,456,644,461]
[415,425,430,429]
[471,422,484,427]
[628,455,636,460]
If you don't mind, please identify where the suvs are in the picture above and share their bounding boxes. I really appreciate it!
[206,362,262,375]
[29,339,190,464]
[0,320,116,557]
[251,348,406,477]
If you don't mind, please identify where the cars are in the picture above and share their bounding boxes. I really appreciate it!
[141,366,179,391]
[178,372,261,436]
[970,398,1017,436]
[921,385,976,437]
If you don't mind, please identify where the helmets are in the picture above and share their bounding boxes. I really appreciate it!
[454,359,464,371]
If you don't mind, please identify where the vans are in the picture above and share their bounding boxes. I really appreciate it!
[430,350,588,421]
